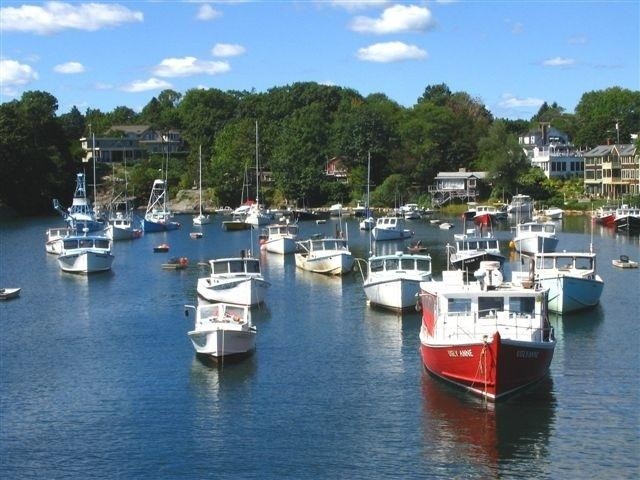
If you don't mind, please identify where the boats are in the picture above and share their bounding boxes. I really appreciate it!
[1,287,24,301]
[412,375,559,469]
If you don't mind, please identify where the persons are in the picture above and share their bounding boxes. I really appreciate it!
[417,239,422,248]
[334,229,345,239]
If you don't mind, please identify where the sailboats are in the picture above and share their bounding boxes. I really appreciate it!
[358,151,378,234]
[87,131,107,225]
[243,120,273,227]
[191,143,213,227]
